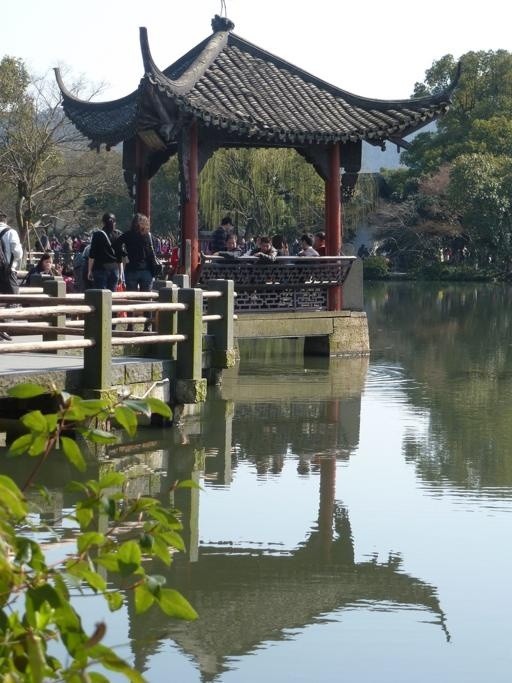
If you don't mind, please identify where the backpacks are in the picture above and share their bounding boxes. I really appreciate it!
[0,227,14,283]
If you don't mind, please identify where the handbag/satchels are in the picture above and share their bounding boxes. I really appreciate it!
[151,252,163,278]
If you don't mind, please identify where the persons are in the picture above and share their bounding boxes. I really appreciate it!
[208,217,326,284]
[25,214,171,332]
[0,213,24,342]
[440,244,491,270]
[358,244,374,256]
